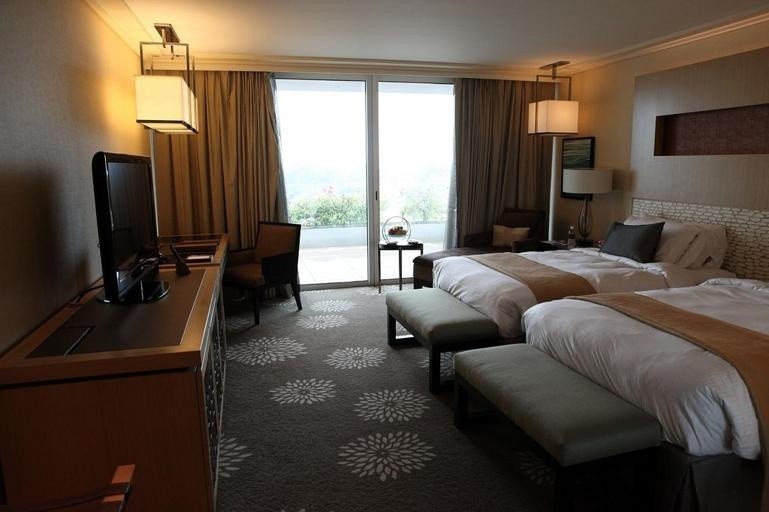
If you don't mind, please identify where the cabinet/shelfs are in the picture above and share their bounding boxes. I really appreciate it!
[5,238,228,508]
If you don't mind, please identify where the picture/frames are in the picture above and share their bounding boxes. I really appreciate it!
[560,136,592,200]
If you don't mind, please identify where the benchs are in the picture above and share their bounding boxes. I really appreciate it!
[452,342,662,509]
[384,287,496,391]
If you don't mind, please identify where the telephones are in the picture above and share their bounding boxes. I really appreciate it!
[168,244,191,277]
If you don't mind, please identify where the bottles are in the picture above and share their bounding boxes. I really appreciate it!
[567,225,577,248]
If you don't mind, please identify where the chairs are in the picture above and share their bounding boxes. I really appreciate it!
[225,220,302,325]
[464,208,545,251]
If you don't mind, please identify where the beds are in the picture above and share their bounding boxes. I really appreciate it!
[522,198,769,462]
[432,198,736,338]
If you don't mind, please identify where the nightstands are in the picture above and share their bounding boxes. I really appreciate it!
[544,238,603,250]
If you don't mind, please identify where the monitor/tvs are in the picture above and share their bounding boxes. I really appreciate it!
[89,148,172,308]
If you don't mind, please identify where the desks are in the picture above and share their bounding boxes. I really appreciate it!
[377,241,423,290]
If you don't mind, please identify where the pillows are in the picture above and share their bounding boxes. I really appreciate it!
[600,221,665,263]
[493,224,529,246]
[623,214,728,269]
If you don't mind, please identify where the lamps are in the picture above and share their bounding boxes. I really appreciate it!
[562,169,614,237]
[134,23,200,136]
[528,59,580,135]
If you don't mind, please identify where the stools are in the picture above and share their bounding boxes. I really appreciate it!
[413,245,490,289]
